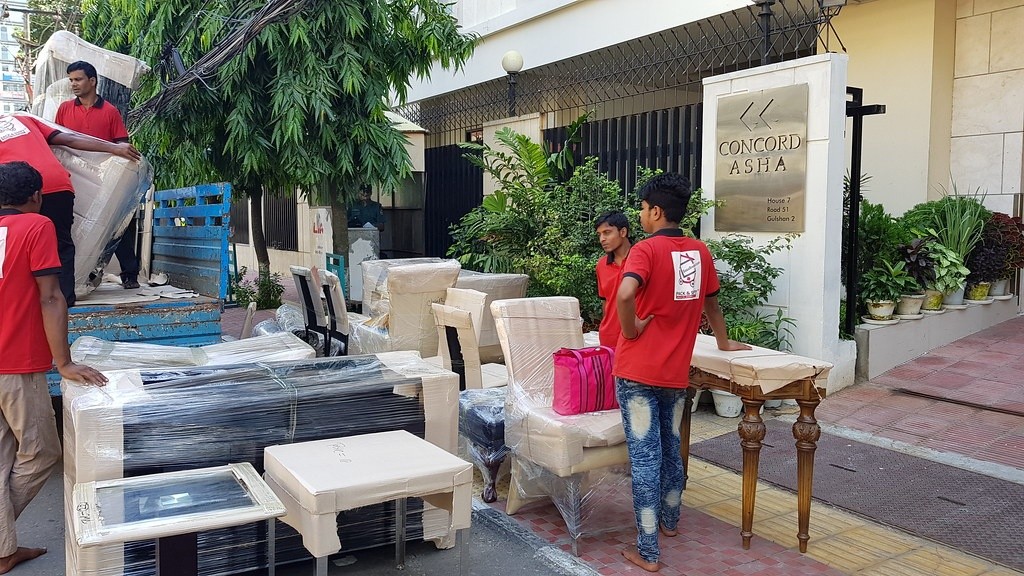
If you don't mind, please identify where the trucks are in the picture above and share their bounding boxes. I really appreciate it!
[44,183,232,398]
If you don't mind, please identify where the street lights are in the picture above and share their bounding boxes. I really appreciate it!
[501,49,525,118]
[750,0,776,66]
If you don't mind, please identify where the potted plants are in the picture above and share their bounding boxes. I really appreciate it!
[692,307,798,417]
[861,170,1024,324]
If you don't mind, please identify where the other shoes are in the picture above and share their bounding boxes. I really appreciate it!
[122,274,140,289]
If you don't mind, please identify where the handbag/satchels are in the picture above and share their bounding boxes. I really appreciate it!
[552,345,619,415]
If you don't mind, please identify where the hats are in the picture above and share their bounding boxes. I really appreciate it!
[359,181,372,196]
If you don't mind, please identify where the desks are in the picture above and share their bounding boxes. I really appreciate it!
[680,335,834,553]
[264,429,473,576]
[72,462,289,576]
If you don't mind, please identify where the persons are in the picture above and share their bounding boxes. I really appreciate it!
[611,173,753,572]
[595,210,636,353]
[53,60,141,290]
[0,160,109,576]
[0,114,141,309]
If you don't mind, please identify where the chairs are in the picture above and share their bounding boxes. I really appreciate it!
[289,256,629,557]
[221,301,257,343]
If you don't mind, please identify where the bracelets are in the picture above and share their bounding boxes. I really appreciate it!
[622,330,638,339]
